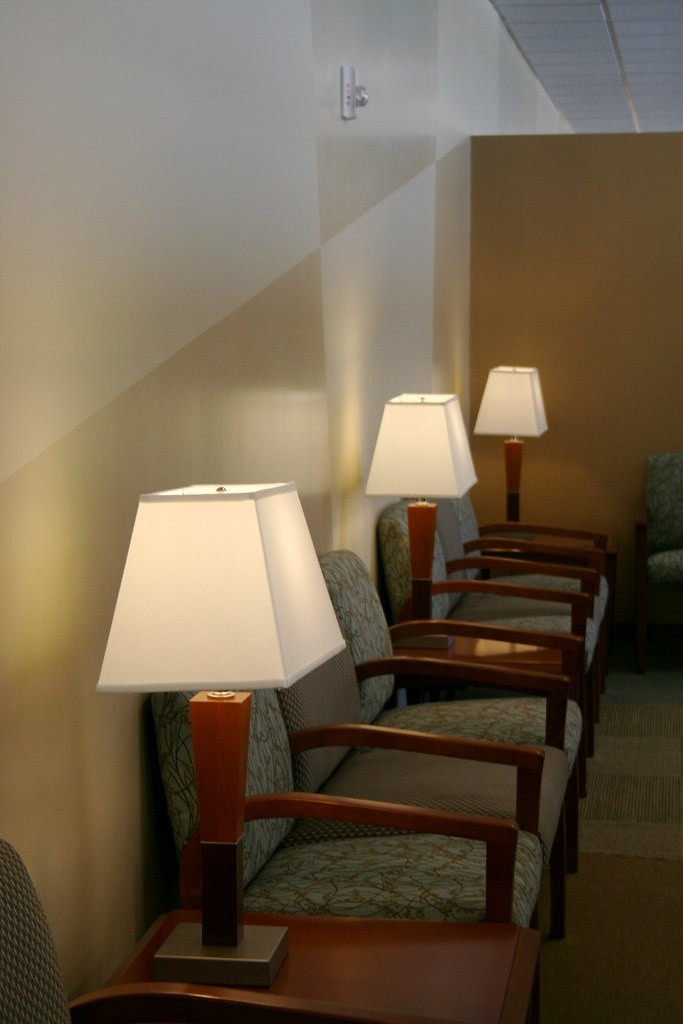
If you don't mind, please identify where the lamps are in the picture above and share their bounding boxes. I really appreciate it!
[472,360,553,539]
[367,387,481,649]
[89,480,356,994]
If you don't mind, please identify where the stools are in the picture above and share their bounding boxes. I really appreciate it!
[99,908,545,1024]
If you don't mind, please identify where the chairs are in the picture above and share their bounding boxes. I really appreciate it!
[0,482,614,1024]
[630,451,683,677]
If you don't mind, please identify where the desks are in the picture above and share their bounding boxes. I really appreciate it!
[382,628,577,673]
[470,523,621,638]
